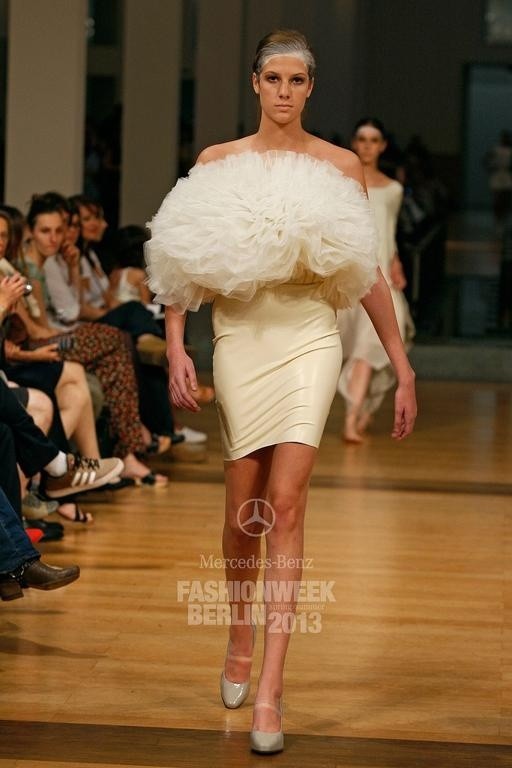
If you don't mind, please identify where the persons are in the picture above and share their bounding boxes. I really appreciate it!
[335,118,416,442]
[375,130,454,247]
[135,25,416,757]
[481,130,511,218]
[1,188,217,602]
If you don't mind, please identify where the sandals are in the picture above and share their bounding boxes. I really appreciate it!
[135,384,215,488]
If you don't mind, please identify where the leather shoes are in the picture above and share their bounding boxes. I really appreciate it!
[21,451,135,543]
[250,696,284,752]
[220,619,256,709]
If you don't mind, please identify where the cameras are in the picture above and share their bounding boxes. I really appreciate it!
[20,283,33,298]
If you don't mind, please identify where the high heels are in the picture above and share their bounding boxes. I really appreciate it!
[0,556,80,602]
